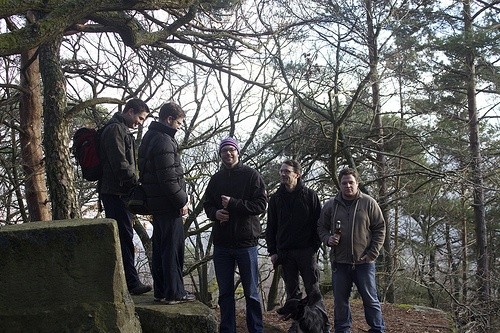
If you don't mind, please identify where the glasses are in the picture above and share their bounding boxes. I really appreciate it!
[220,148,237,153]
[279,169,294,174]
[340,180,356,185]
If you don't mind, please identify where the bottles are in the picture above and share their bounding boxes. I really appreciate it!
[333,220,341,244]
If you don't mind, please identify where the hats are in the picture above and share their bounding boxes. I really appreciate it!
[218,137,240,155]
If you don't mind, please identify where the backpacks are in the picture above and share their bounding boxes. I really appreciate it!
[72,120,128,182]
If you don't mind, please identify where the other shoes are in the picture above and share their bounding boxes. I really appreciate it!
[169,293,196,304]
[129,282,152,293]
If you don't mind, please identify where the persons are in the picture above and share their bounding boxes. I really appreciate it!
[265,161,325,308]
[138,102,196,304]
[317,168,386,333]
[99,99,152,295]
[203,138,267,333]
